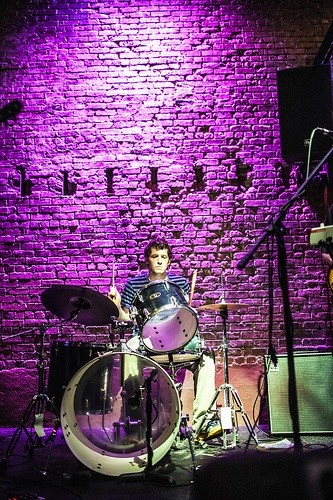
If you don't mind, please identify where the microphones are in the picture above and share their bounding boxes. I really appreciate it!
[110,316,116,338]
[128,368,159,410]
[237,231,269,269]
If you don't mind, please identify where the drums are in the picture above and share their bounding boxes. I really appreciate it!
[59,351,183,476]
[45,339,119,415]
[136,325,205,362]
[129,279,199,354]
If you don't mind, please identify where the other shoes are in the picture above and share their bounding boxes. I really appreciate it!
[197,420,221,441]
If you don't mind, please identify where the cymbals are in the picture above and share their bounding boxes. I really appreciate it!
[40,285,119,326]
[197,303,250,311]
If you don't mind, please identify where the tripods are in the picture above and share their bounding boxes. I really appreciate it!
[192,308,260,449]
[0,323,61,470]
[118,383,177,485]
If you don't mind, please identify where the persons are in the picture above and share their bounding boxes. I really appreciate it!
[108,241,222,440]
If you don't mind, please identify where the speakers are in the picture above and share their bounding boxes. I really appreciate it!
[264,353,332,436]
